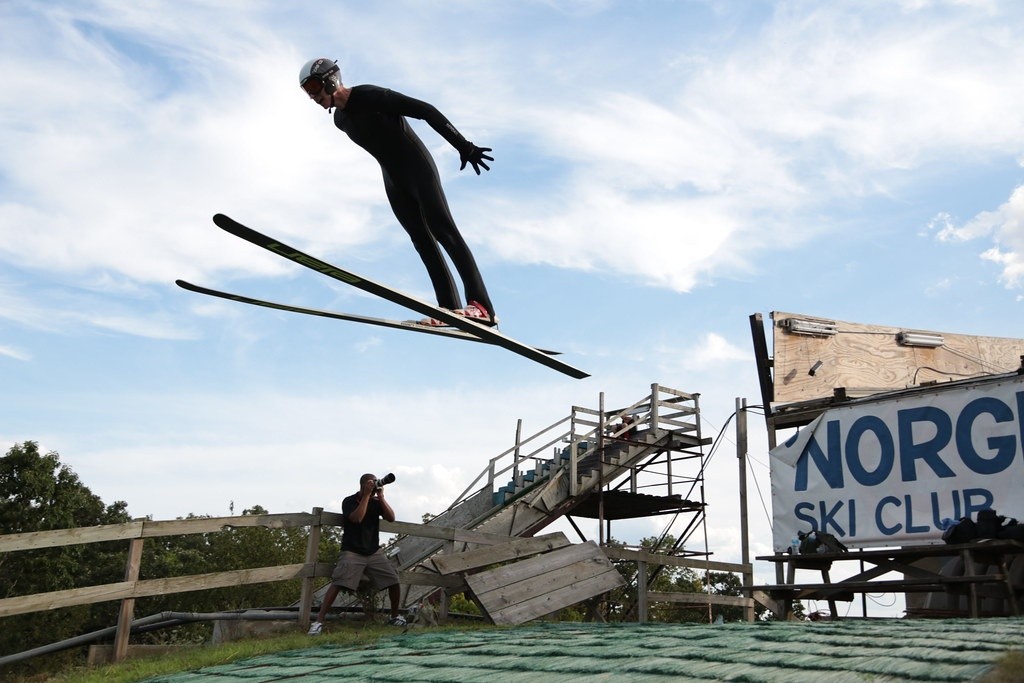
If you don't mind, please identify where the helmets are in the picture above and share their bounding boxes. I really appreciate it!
[299,58,342,96]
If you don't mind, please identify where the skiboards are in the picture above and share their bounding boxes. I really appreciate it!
[173,211,593,381]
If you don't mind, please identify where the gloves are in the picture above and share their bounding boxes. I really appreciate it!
[460,143,495,174]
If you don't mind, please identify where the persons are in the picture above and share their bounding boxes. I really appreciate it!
[307,473,411,635]
[300,57,499,331]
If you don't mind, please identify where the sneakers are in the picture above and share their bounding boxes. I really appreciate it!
[451,300,499,328]
[405,306,458,328]
[308,622,323,635]
[389,614,407,626]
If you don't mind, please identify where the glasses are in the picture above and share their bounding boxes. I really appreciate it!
[301,65,339,96]
